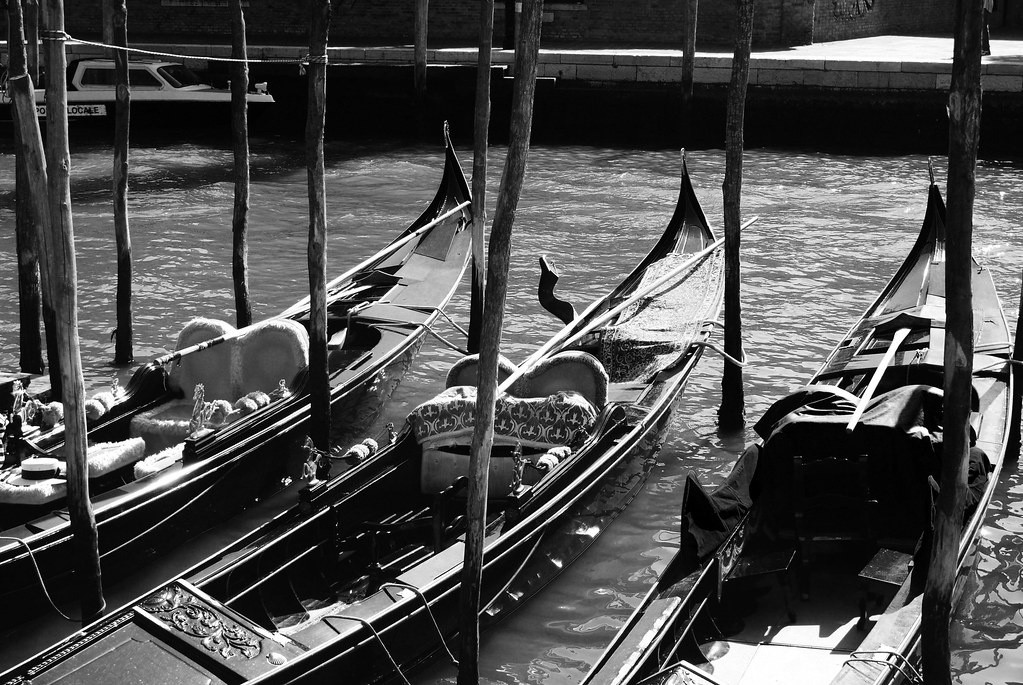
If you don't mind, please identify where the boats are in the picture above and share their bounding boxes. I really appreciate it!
[579,158,1013,685]
[0,61,276,148]
[0,118,476,613]
[0,147,727,685]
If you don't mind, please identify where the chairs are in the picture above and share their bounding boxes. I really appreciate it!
[791,454,875,605]
[361,475,472,597]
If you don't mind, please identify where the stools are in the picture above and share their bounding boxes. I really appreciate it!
[857,549,912,629]
[727,546,799,625]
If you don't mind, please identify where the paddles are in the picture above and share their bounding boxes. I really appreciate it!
[281,200,471,315]
[495,295,607,398]
[154,282,379,367]
[846,328,912,433]
[533,216,758,365]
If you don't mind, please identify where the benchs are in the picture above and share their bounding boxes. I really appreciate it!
[447,348,608,406]
[753,383,974,445]
[123,318,309,445]
